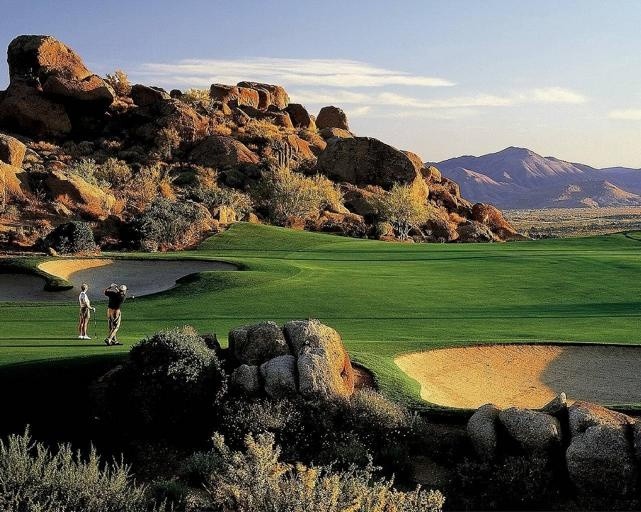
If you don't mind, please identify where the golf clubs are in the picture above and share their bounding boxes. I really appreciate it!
[93,307,98,340]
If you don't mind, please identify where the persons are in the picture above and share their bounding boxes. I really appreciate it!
[78,282,95,339]
[104,283,128,345]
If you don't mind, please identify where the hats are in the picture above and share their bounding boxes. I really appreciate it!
[119,284,128,290]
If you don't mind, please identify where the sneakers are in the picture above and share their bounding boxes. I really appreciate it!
[113,343,123,345]
[105,338,111,345]
[83,335,91,339]
[78,335,82,339]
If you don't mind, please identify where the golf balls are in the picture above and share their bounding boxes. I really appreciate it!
[132,295,135,299]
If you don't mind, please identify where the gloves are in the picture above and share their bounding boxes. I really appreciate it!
[110,283,117,288]
